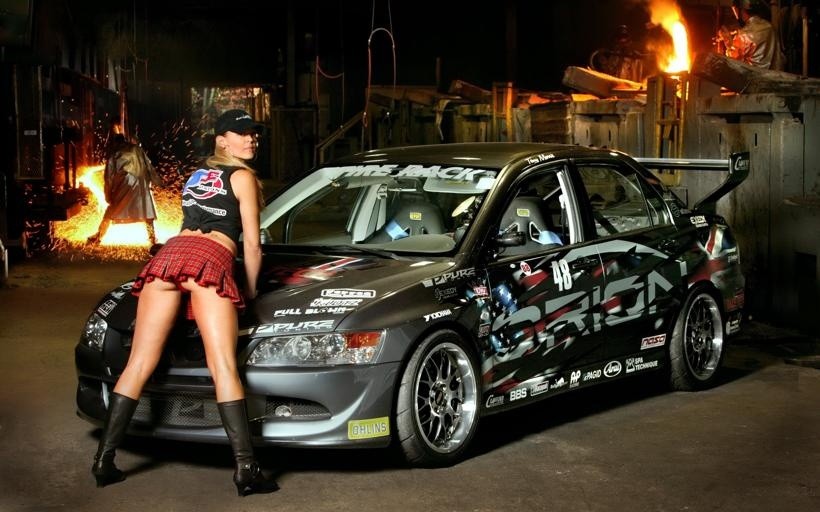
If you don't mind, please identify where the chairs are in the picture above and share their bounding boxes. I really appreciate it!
[497,195,562,256]
[355,201,445,245]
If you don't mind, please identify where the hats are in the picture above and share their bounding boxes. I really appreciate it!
[214,108,263,136]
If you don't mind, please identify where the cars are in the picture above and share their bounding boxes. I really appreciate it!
[72,137,751,470]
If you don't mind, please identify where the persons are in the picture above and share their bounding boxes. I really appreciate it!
[711,0,790,73]
[84,107,284,495]
[82,129,161,251]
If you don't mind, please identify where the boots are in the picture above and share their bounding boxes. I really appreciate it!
[217,397,281,496]
[90,391,139,487]
